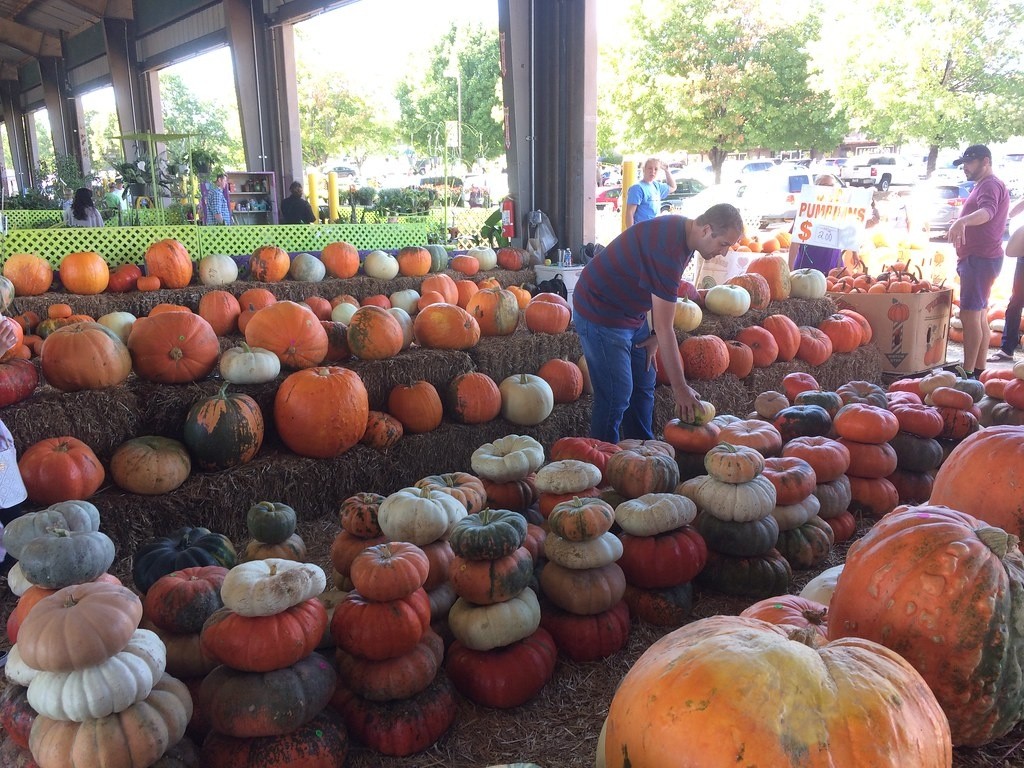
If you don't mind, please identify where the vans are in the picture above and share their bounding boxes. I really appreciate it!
[728,160,775,185]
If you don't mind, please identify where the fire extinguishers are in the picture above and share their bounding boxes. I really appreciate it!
[501,193,515,238]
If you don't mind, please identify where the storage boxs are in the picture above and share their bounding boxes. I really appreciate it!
[826,290,954,373]
[696,252,789,291]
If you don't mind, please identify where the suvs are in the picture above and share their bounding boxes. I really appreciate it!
[658,177,709,212]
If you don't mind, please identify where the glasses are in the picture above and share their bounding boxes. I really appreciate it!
[960,153,976,158]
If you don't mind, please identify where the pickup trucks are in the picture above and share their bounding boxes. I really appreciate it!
[840,153,923,191]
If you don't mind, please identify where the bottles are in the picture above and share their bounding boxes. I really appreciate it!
[565,248,571,266]
[247,178,266,192]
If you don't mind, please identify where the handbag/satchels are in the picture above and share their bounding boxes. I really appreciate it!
[0,421,28,509]
[526,223,542,265]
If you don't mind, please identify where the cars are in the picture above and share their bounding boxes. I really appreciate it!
[778,159,846,181]
[596,186,622,212]
[662,162,713,176]
[912,185,969,231]
[928,153,1024,196]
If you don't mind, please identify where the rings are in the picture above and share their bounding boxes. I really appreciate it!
[957,236,961,238]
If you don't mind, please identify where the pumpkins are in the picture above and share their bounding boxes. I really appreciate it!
[0,225,1024,768]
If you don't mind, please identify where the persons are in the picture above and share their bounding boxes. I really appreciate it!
[625,158,676,230]
[64,188,105,228]
[206,174,230,226]
[986,195,1024,362]
[282,182,317,225]
[103,179,128,212]
[0,320,18,359]
[942,145,1008,379]
[788,174,860,278]
[573,203,743,444]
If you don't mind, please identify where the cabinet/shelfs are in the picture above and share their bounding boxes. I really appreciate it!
[535,265,584,322]
[218,170,279,226]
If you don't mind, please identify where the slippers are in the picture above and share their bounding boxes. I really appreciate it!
[986,350,1013,362]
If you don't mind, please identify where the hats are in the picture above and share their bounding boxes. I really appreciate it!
[953,145,990,165]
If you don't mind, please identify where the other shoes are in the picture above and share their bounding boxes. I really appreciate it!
[953,368,977,380]
[975,368,985,379]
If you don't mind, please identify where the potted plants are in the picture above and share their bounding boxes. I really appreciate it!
[191,151,215,181]
[318,188,437,223]
[108,149,179,199]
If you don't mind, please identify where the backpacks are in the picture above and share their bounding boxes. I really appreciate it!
[537,273,567,302]
